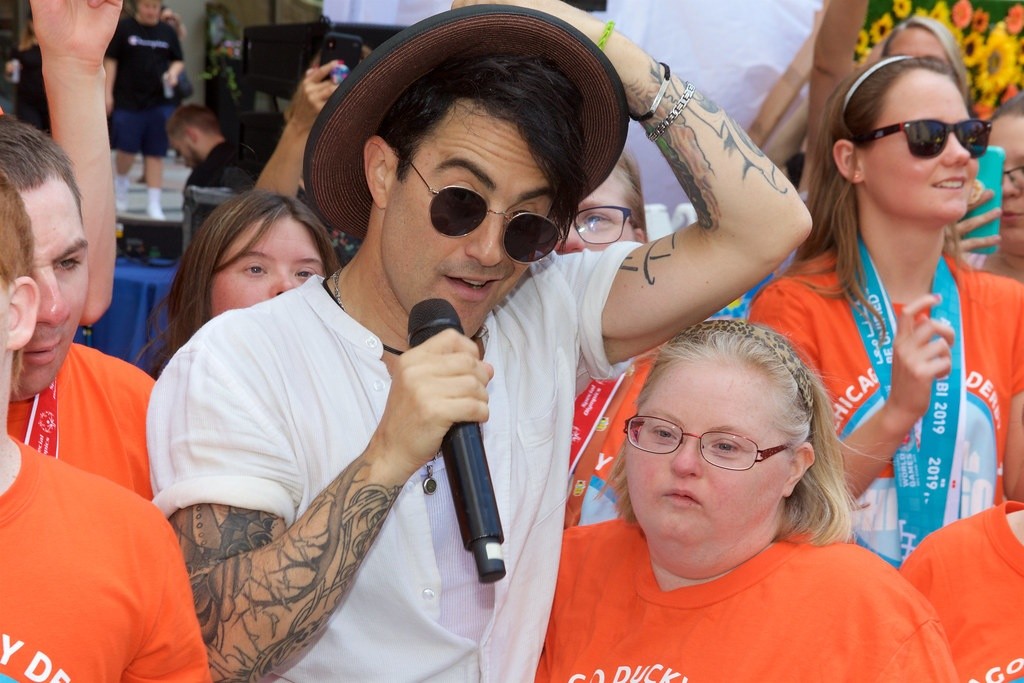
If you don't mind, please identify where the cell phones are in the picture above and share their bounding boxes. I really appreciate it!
[320,32,362,85]
[959,145,1005,255]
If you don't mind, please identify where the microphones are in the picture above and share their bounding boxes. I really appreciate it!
[407,297,508,586]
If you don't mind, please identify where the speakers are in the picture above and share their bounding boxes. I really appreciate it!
[235,21,408,100]
[239,113,288,180]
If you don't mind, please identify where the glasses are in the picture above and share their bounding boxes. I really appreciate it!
[1003,165,1024,191]
[389,146,563,265]
[562,206,640,245]
[850,120,992,159]
[624,412,791,472]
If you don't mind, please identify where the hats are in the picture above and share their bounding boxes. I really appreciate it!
[303,4,630,240]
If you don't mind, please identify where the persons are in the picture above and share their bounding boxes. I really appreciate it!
[556,1,1024,530]
[746,54,1024,571]
[0,172,212,683]
[899,500,1024,683]
[0,0,371,501]
[533,319,958,683]
[144,1,814,683]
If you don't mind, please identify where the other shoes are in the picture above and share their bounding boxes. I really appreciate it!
[146,206,164,219]
[114,184,130,212]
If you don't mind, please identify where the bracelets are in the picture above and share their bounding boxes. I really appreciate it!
[629,61,671,121]
[596,20,616,53]
[645,80,696,143]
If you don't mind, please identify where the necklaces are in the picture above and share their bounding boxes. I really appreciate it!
[322,266,443,495]
